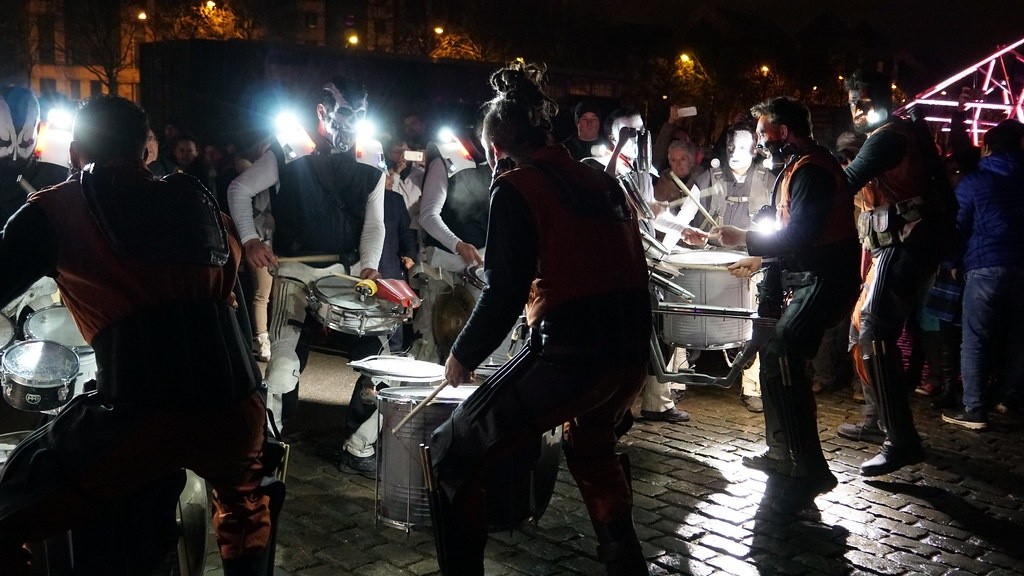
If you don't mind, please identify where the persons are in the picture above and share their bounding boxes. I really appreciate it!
[711,96,858,495]
[0,94,286,576]
[0,68,1024,477]
[422,62,654,576]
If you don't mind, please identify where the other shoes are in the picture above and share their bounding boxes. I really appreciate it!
[255,334,272,362]
[772,463,838,511]
[742,454,792,474]
[915,383,942,396]
[860,431,925,476]
[837,415,887,444]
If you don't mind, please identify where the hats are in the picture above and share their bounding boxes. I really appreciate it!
[574,99,602,126]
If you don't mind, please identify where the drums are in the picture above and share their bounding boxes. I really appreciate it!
[658,248,755,351]
[464,263,490,301]
[0,430,76,576]
[636,130,653,174]
[642,250,682,280]
[0,310,18,353]
[659,302,756,319]
[638,225,670,261]
[647,268,695,303]
[375,384,535,532]
[0,338,80,414]
[625,171,656,219]
[346,353,447,384]
[307,275,406,334]
[22,304,97,415]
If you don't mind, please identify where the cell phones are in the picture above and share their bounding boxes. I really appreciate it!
[677,107,697,117]
[404,150,423,161]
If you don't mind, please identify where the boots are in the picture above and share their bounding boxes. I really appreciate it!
[930,365,959,409]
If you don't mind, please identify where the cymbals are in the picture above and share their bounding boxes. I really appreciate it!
[430,284,476,352]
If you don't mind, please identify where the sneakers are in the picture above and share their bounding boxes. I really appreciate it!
[940,409,990,431]
[811,381,835,393]
[640,407,691,422]
[739,394,764,413]
[338,450,383,480]
[671,389,685,404]
[853,388,866,404]
